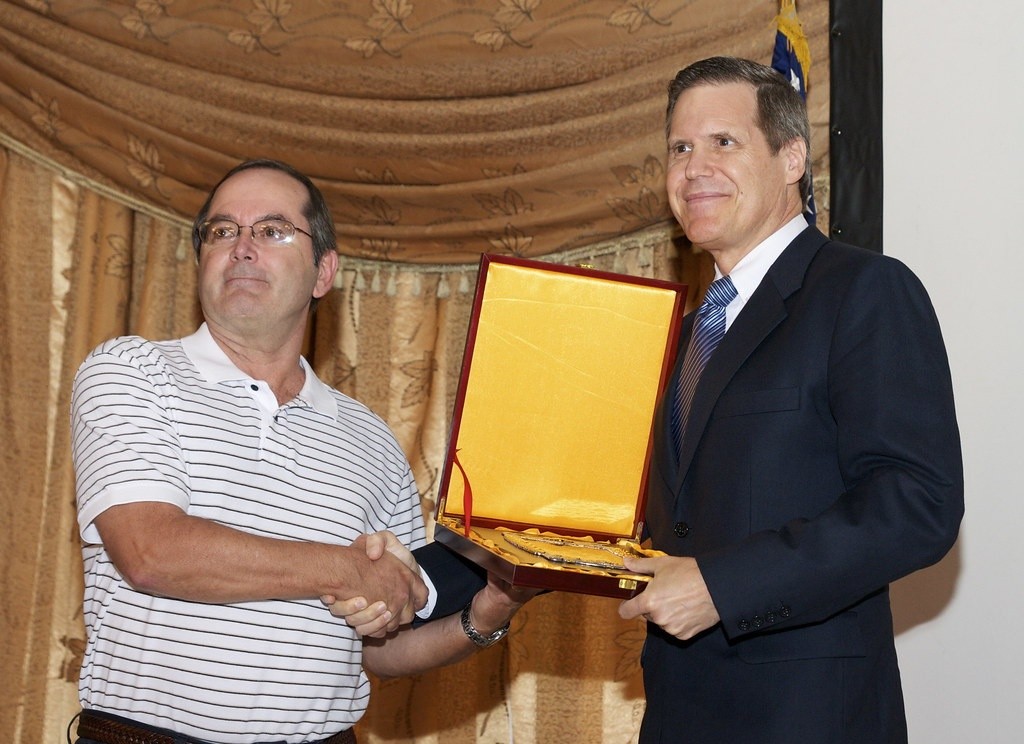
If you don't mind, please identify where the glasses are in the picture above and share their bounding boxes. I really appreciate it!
[198,220,313,245]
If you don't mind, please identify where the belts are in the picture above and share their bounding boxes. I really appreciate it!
[77,714,358,744]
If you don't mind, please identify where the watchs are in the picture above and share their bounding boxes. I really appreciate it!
[460,602,511,649]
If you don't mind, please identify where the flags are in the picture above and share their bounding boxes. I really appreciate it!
[769,15,816,225]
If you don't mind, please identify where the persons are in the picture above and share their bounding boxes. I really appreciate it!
[72,159,544,744]
[320,58,968,743]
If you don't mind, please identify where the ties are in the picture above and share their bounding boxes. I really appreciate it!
[672,276,738,473]
